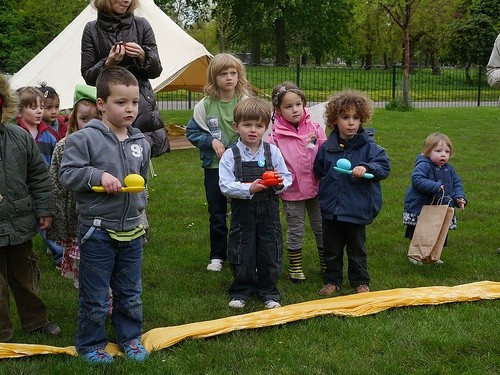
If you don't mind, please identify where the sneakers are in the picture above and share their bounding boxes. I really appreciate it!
[228,298,246,308]
[207,258,223,272]
[264,298,281,308]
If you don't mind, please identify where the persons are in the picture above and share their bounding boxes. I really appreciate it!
[266,81,328,283]
[186,55,253,272]
[218,95,292,309]
[313,89,390,295]
[81,0,171,244]
[0,73,68,343]
[57,66,150,363]
[403,132,468,266]
[486,33,500,253]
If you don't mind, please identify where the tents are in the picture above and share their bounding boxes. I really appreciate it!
[9,0,272,110]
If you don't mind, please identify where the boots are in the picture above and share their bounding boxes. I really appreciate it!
[288,248,306,282]
[318,247,327,277]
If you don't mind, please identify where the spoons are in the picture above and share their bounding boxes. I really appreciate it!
[334,167,374,178]
[92,185,146,192]
[246,179,282,186]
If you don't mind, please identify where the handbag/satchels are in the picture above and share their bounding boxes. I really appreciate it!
[408,187,454,262]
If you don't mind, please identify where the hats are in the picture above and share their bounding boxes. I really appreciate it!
[74,84,98,105]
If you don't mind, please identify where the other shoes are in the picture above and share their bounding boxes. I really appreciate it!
[120,338,147,362]
[319,284,337,295]
[81,349,114,365]
[40,323,61,336]
[409,256,423,266]
[355,283,370,293]
[432,259,444,264]
